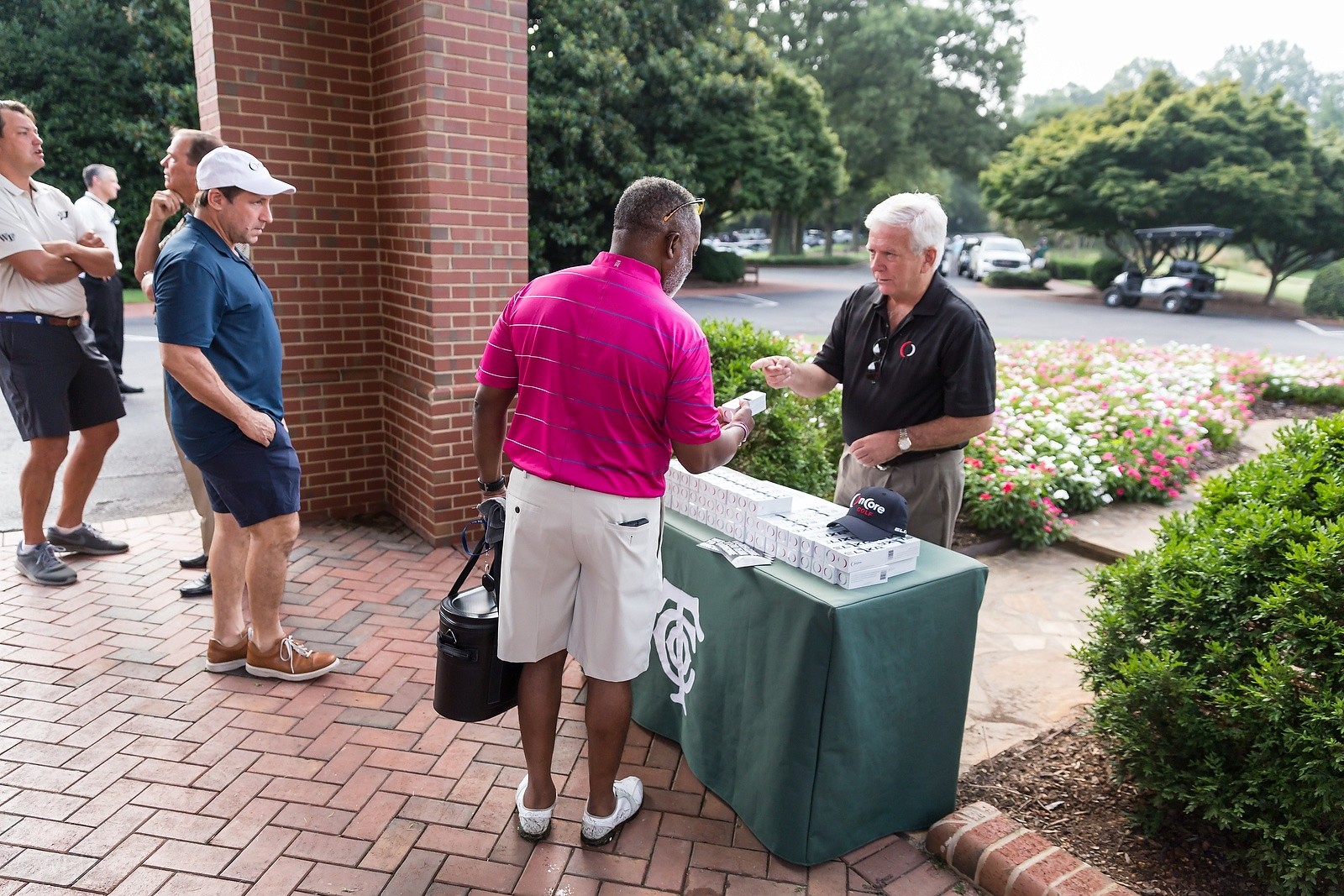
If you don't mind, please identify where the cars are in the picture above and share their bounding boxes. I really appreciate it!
[720,233,737,242]
[708,233,723,242]
[969,237,1031,281]
[804,233,820,245]
[734,228,767,241]
[832,231,852,242]
[958,238,982,275]
[836,230,863,238]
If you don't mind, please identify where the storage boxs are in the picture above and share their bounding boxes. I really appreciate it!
[664,458,919,590]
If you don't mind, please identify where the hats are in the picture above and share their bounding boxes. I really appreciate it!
[196,145,297,195]
[827,487,909,541]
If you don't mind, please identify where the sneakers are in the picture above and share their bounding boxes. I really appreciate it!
[246,632,339,680]
[14,540,76,585]
[205,625,252,672]
[48,522,129,555]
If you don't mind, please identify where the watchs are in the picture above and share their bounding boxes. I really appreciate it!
[897,428,911,452]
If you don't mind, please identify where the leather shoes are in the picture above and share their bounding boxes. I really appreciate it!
[580,776,643,846]
[180,572,212,596]
[179,555,210,568]
[117,377,144,393]
[515,772,557,840]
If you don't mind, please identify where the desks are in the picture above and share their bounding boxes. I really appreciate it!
[630,479,990,867]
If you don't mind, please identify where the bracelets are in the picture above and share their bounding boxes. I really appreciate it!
[142,270,154,276]
[724,421,750,447]
[476,475,505,491]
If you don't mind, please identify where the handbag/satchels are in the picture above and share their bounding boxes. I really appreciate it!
[434,530,522,722]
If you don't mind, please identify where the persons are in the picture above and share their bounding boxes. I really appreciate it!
[0,100,129,586]
[74,163,145,404]
[133,128,250,597]
[750,192,998,549]
[152,145,340,682]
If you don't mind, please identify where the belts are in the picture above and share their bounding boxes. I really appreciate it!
[0,311,82,327]
[875,449,950,470]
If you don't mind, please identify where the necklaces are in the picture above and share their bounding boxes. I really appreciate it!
[472,176,756,847]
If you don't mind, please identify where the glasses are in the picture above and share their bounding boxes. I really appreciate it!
[664,197,706,221]
[867,335,889,384]
